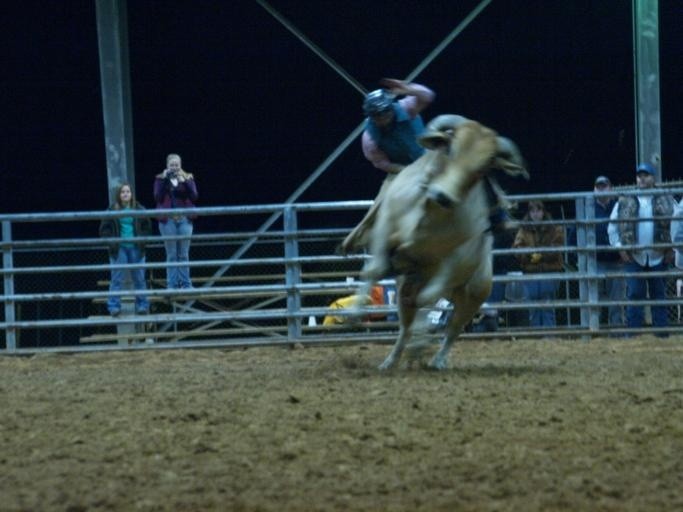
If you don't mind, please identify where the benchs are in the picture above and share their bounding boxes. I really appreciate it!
[79,270,363,343]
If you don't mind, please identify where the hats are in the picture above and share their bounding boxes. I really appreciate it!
[595,177,609,185]
[363,89,392,115]
[636,163,655,174]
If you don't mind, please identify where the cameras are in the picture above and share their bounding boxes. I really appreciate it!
[167,170,179,180]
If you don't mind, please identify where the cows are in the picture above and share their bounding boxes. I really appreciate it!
[334,115,531,374]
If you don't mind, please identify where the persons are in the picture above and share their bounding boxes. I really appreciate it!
[152,152,195,289]
[473,163,682,338]
[98,181,153,316]
[359,75,510,277]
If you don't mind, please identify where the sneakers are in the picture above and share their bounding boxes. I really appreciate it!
[496,219,520,230]
